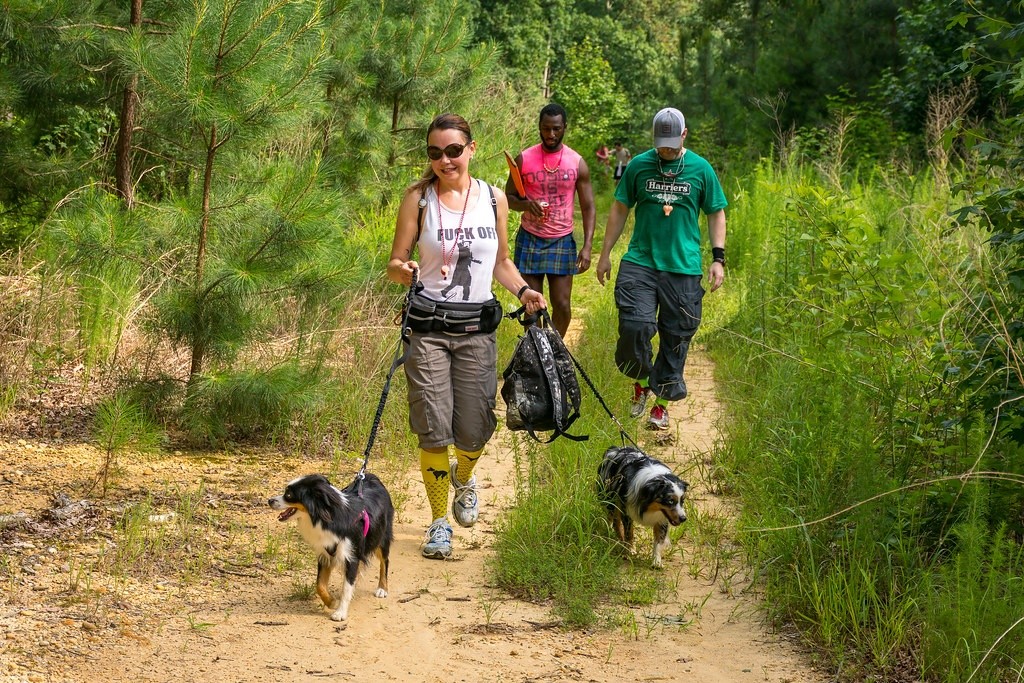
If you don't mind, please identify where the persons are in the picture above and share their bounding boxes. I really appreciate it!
[505,103,594,339]
[608,142,631,185]
[387,113,548,558]
[596,146,609,164]
[596,107,728,431]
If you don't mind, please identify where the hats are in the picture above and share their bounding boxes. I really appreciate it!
[652,107,685,149]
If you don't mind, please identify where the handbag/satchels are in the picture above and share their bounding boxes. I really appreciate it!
[402,294,502,334]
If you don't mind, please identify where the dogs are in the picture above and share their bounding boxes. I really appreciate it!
[268,474,395,622]
[597,443,689,570]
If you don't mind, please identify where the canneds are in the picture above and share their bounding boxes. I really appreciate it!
[540,202,550,222]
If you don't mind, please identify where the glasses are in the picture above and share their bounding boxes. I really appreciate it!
[427,142,467,160]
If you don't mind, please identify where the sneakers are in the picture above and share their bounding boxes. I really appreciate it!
[419,518,454,560]
[628,383,650,419]
[645,406,671,431]
[449,460,480,528]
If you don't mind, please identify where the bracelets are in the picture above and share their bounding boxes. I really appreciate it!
[517,286,530,299]
[712,247,725,266]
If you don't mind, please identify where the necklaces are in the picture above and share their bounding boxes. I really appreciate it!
[658,156,682,216]
[541,146,563,173]
[656,152,684,177]
[437,175,472,280]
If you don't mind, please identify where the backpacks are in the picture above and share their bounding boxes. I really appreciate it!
[502,310,590,442]
